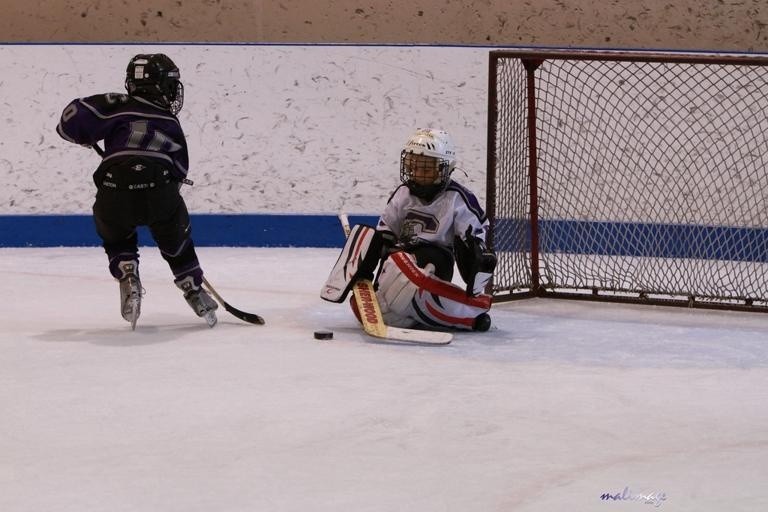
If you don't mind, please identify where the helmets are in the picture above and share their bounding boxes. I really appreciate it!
[126,53,186,117]
[398,128,463,201]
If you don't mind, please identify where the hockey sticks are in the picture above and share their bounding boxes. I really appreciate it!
[338,210,453,343]
[93,140,266,325]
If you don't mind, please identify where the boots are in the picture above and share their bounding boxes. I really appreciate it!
[109,253,143,331]
[171,270,219,328]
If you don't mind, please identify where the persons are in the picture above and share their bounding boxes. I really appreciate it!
[319,126,499,334]
[53,51,219,325]
